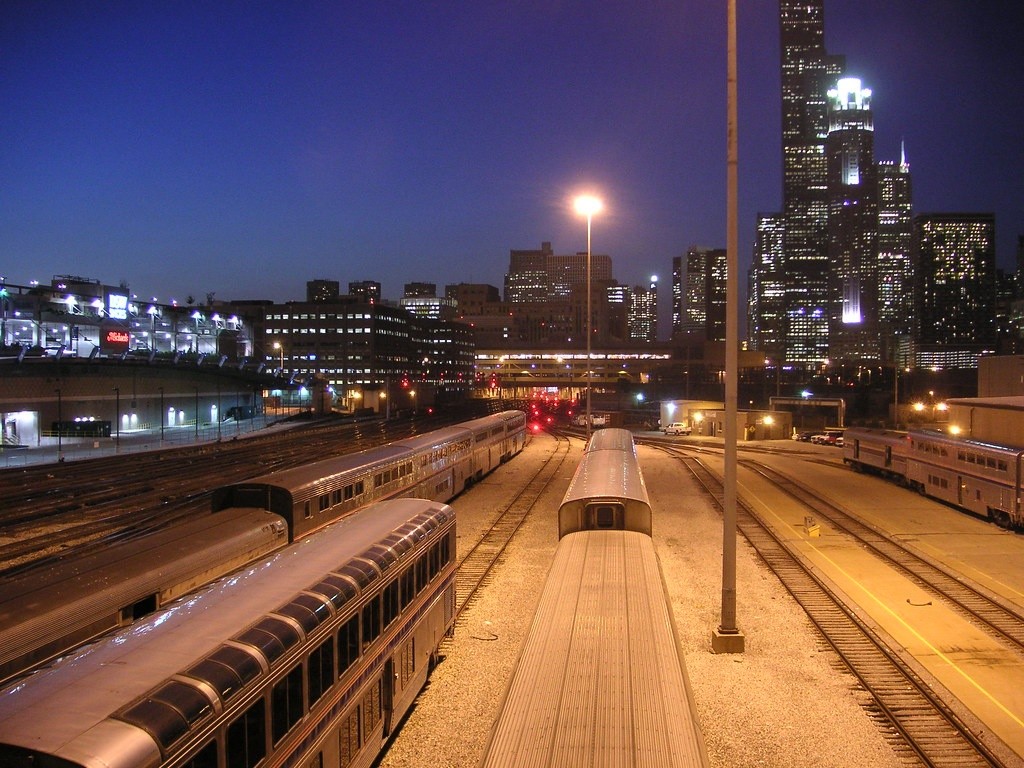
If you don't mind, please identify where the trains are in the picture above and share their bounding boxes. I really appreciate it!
[479,424,708,768]
[840,423,1024,535]
[0,406,527,680]
[0,496,457,768]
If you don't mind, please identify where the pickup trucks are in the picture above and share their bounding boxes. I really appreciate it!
[664,423,692,436]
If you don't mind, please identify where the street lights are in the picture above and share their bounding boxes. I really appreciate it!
[192,386,200,442]
[235,389,241,436]
[274,342,284,379]
[572,193,601,448]
[113,387,121,453]
[157,387,165,448]
[214,386,222,441]
[52,388,64,461]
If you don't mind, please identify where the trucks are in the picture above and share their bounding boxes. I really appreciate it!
[577,414,605,429]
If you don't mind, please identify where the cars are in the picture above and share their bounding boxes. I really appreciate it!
[792,430,843,447]
